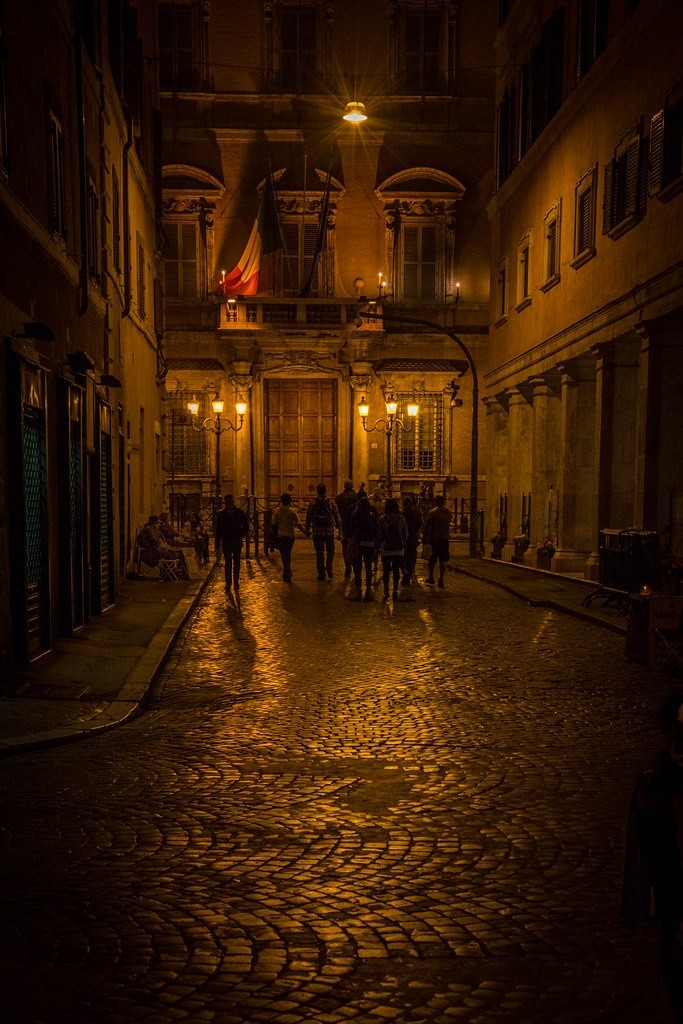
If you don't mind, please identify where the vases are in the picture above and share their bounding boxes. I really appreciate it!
[536,555,552,572]
[492,544,501,555]
[514,547,525,557]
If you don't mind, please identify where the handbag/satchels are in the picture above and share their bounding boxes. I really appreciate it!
[268,521,279,540]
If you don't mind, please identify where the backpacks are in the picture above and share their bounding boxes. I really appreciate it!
[310,498,334,527]
[382,514,407,551]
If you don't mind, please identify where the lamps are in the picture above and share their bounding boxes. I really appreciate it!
[342,101,368,124]
[14,321,56,345]
[95,370,122,392]
[64,350,95,375]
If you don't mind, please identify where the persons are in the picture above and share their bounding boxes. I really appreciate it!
[334,479,452,603]
[623,692,683,1024]
[137,510,208,580]
[272,493,310,583]
[215,494,248,591]
[305,484,342,580]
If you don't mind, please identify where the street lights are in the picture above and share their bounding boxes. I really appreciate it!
[187,385,246,508]
[359,394,419,495]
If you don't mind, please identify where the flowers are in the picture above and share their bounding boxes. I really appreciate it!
[489,529,507,550]
[512,534,530,548]
[535,538,558,558]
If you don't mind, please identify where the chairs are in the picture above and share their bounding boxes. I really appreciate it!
[135,545,160,580]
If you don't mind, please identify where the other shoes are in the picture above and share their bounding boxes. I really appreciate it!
[425,578,436,584]
[354,587,362,597]
[384,589,390,598]
[344,569,351,581]
[438,579,445,588]
[225,586,231,596]
[412,580,421,586]
[365,586,374,597]
[318,574,326,580]
[400,579,410,586]
[325,566,334,579]
[281,573,293,583]
[393,591,400,600]
[234,582,239,591]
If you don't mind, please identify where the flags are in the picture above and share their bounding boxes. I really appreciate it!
[218,166,285,296]
[295,163,333,298]
